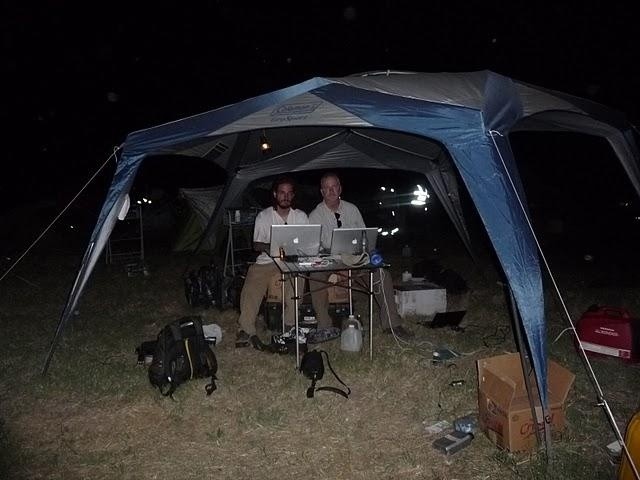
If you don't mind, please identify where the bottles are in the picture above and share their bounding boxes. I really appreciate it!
[279,247,285,260]
[369,247,382,265]
[235,210,241,222]
[340,314,364,353]
[358,230,369,254]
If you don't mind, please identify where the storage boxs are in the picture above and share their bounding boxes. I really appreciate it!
[474,351,577,454]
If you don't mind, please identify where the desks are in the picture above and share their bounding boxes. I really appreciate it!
[266,249,395,373]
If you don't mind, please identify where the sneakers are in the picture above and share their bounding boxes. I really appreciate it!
[383,326,409,338]
[310,327,339,343]
[235,331,250,348]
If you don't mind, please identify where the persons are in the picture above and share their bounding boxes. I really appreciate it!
[233,177,342,350]
[307,172,415,343]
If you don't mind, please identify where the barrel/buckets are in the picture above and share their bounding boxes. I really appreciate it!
[340,314,364,353]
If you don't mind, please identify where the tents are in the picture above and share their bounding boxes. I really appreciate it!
[37,69,640,480]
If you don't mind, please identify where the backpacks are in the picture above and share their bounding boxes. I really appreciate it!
[148,315,218,397]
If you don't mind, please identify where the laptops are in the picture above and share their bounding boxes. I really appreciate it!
[329,228,378,254]
[270,224,321,257]
[417,311,466,329]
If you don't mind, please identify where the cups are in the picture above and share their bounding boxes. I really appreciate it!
[402,273,408,281]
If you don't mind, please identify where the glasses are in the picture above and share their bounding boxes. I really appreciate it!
[335,213,342,228]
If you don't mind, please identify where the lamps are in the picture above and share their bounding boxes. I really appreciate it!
[256,128,273,154]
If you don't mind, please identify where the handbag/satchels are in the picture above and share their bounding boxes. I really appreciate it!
[300,349,350,399]
